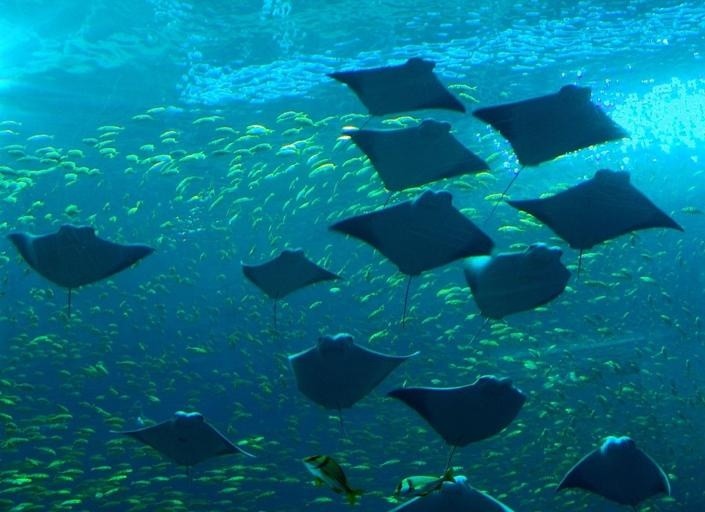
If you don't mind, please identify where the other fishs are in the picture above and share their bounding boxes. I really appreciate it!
[0,55,705,512]
